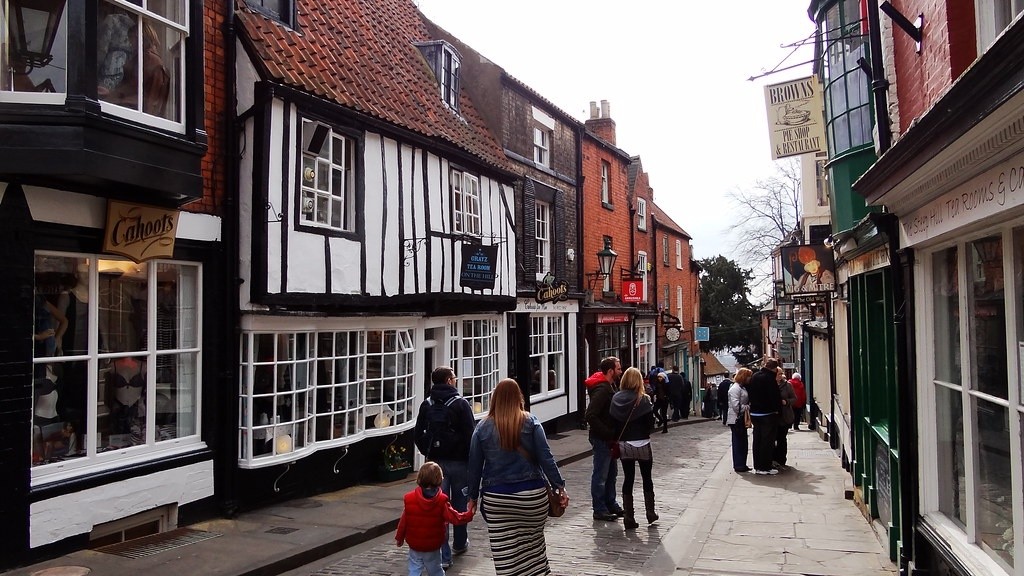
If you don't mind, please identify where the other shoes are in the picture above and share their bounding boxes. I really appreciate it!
[758,470,779,475]
[452,538,469,553]
[657,418,662,427]
[607,505,626,516]
[593,512,617,520]
[439,560,453,567]
[736,467,753,473]
[661,430,668,434]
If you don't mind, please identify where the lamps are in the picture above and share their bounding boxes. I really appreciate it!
[1,0,67,76]
[586,238,618,291]
[374,414,390,428]
[824,229,850,247]
[276,435,292,455]
[474,402,482,414]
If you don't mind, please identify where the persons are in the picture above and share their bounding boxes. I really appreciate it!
[725,358,796,474]
[788,373,806,431]
[34,364,58,424]
[395,462,477,576]
[703,373,736,425]
[413,365,475,568]
[97,23,164,114]
[98,3,136,88]
[45,422,74,457]
[109,356,147,416]
[641,362,692,434]
[34,296,68,378]
[55,263,111,449]
[531,370,557,392]
[610,367,659,530]
[468,379,569,576]
[584,357,623,521]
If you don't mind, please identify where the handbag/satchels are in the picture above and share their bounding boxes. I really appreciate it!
[784,406,794,424]
[546,491,565,518]
[744,407,751,428]
[610,443,620,459]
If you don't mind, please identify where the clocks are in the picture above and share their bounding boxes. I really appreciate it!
[665,328,680,342]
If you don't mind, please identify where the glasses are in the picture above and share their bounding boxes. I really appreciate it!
[450,377,458,381]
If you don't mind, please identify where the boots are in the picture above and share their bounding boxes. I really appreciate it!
[645,493,659,524]
[623,495,639,530]
